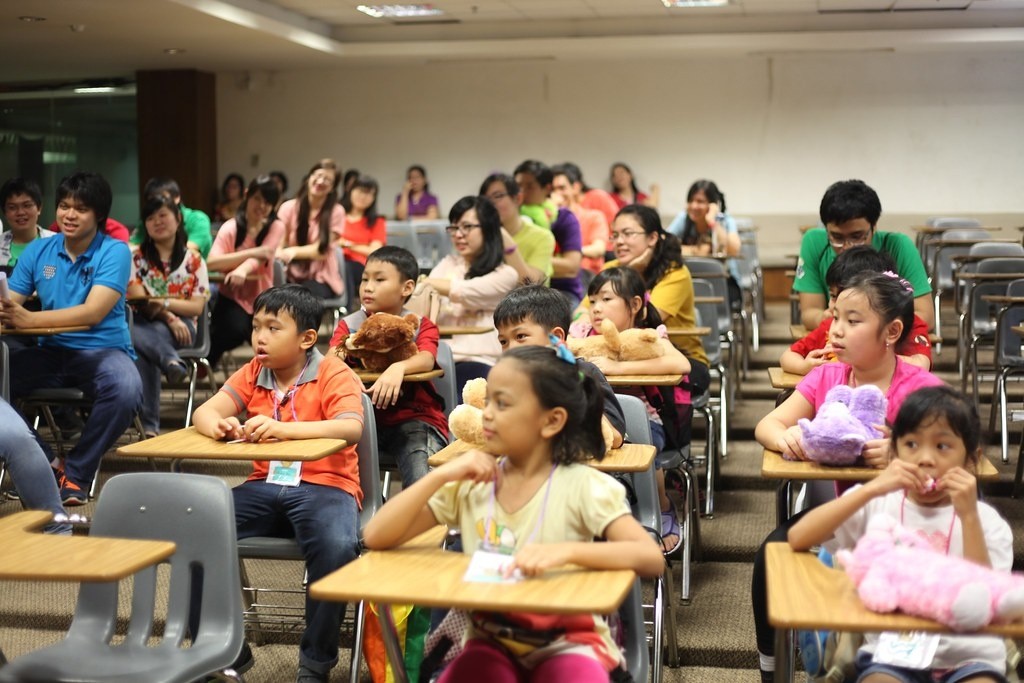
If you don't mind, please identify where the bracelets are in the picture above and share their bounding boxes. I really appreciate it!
[504,244,518,254]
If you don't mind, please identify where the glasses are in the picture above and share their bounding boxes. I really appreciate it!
[489,191,508,201]
[829,225,871,249]
[608,229,645,241]
[446,224,481,236]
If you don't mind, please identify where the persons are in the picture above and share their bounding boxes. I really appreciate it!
[787,386,1024,683]
[779,245,933,375]
[1,176,59,267]
[754,269,945,469]
[792,179,937,334]
[665,179,741,257]
[326,245,451,491]
[190,285,365,683]
[393,165,440,220]
[570,204,711,399]
[567,265,692,556]
[0,159,661,683]
[0,168,145,504]
[493,275,627,449]
[362,345,665,683]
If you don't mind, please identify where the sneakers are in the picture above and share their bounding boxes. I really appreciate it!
[3,457,65,499]
[59,474,87,505]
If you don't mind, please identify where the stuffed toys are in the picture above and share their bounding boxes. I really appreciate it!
[567,318,662,362]
[448,377,614,459]
[782,383,889,467]
[335,312,420,374]
[835,510,1024,634]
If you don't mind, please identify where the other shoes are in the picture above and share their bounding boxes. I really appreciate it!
[665,477,706,512]
[166,364,187,389]
[194,642,254,683]
[295,659,329,683]
[196,362,207,379]
[139,431,156,441]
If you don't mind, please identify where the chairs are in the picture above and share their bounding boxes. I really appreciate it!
[0,218,1024,683]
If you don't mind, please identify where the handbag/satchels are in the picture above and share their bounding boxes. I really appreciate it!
[364,599,431,683]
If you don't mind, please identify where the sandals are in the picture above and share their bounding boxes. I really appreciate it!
[660,492,683,555]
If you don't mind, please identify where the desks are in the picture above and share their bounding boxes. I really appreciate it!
[763,542,1024,683]
[662,325,713,336]
[602,373,690,386]
[116,424,346,463]
[350,368,447,381]
[425,434,659,541]
[0,510,179,587]
[434,326,496,334]
[766,366,809,389]
[762,434,999,528]
[308,534,652,683]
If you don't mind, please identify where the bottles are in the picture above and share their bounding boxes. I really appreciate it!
[712,206,727,258]
